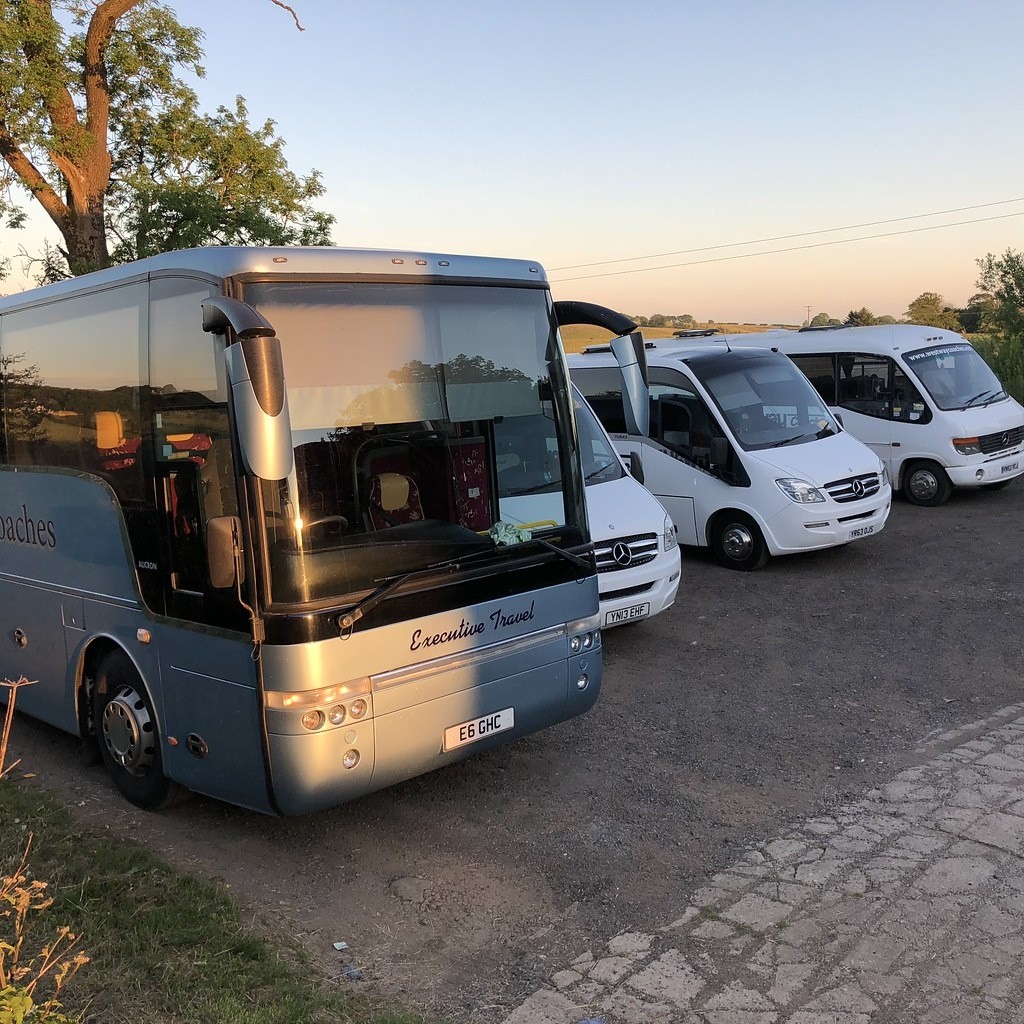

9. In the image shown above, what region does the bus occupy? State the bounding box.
[452,372,682,632]
[670,324,1024,509]
[558,328,894,572]
[0,249,653,823]
